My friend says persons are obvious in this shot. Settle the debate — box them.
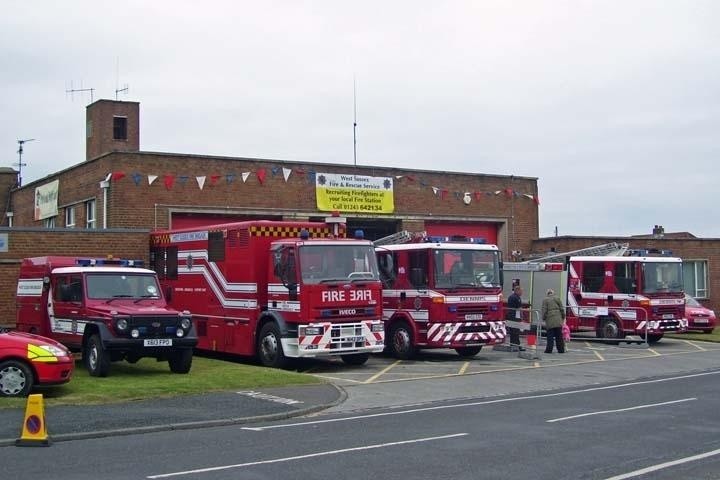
[507,286,532,351]
[542,289,565,353]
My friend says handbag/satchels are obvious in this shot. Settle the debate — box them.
[562,323,570,343]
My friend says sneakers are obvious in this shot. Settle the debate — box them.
[519,348,525,351]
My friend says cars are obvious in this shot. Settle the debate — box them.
[0,326,74,397]
[679,292,716,333]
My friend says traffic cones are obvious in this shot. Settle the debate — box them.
[14,393,53,448]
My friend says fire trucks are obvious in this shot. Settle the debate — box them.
[452,241,684,345]
[374,229,508,358]
[149,215,386,368]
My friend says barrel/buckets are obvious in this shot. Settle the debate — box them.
[526,334,536,344]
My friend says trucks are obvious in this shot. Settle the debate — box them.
[17,252,199,376]
